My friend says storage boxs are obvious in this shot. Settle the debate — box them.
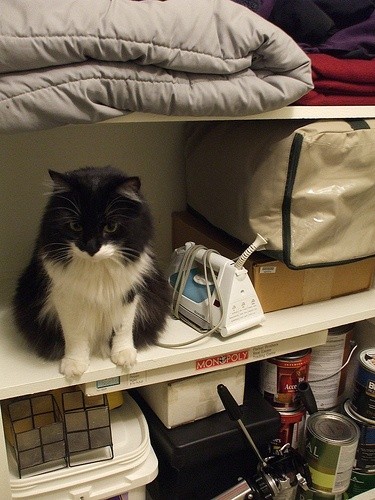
[171,211,375,314]
[136,366,245,429]
[126,389,281,500]
[6,392,159,500]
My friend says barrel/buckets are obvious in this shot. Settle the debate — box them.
[259,329,375,500]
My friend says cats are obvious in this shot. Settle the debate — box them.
[12,164,172,376]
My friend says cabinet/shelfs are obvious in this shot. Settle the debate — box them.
[0,105,375,500]
